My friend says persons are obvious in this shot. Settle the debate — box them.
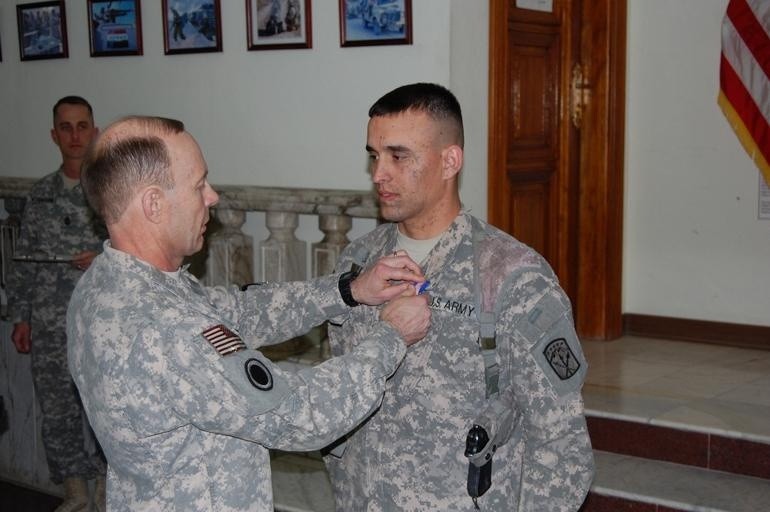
[18,3,298,53]
[62,115,434,511]
[0,94,111,509]
[316,80,596,509]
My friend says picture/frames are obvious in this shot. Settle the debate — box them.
[13,3,69,63]
[338,0,412,48]
[245,0,312,53]
[86,2,146,58]
[161,2,223,55]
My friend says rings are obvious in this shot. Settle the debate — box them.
[77,265,82,268]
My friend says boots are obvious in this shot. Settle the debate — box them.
[54,476,106,512]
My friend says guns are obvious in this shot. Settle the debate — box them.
[464,424,494,499]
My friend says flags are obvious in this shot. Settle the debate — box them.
[716,0,770,188]
